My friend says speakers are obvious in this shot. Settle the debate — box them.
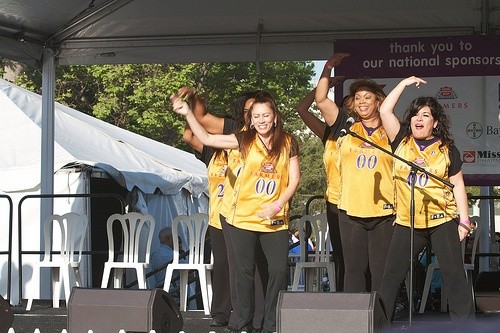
[68,286,184,333]
[0,295,14,333]
[276,289,390,333]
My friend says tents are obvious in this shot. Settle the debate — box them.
[0,0,500,305]
[0,78,209,300]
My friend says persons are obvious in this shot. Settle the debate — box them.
[460,220,470,224]
[170,86,301,333]
[177,236,191,310]
[465,222,477,263]
[146,227,194,305]
[297,53,476,327]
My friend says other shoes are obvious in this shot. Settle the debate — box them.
[209,318,228,326]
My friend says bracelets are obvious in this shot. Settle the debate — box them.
[274,203,281,212]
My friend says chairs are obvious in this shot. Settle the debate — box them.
[162,214,213,316]
[25,211,88,311]
[101,212,155,290]
[405,215,482,314]
[292,212,337,293]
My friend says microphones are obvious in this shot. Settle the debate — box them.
[340,116,355,137]
